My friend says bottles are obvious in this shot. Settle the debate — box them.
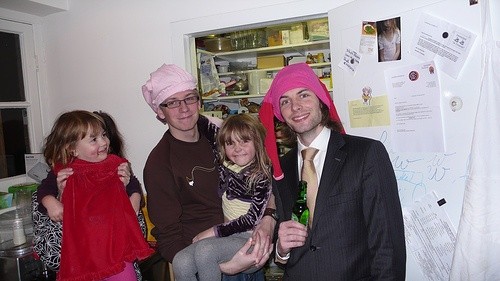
[290,180,310,233]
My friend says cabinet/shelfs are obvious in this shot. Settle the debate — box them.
[197,38,333,100]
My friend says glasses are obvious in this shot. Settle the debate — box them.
[160,96,199,108]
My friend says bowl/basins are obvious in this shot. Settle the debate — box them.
[203,38,232,52]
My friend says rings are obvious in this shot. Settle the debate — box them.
[123,173,127,178]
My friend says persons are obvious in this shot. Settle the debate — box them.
[32,112,148,281]
[270,62,407,281]
[172,114,270,281]
[38,110,155,281]
[143,63,277,281]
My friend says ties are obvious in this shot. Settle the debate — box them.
[301,147,318,230]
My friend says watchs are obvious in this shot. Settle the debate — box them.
[263,206,278,221]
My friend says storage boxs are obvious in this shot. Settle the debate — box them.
[8,183,39,199]
[0,192,13,209]
[266,18,329,44]
[320,78,332,88]
[256,55,286,69]
[259,79,273,94]
[25,153,51,185]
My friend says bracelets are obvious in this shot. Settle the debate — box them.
[274,241,290,261]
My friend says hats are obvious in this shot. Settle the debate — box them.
[142,64,198,113]
[260,63,346,181]
